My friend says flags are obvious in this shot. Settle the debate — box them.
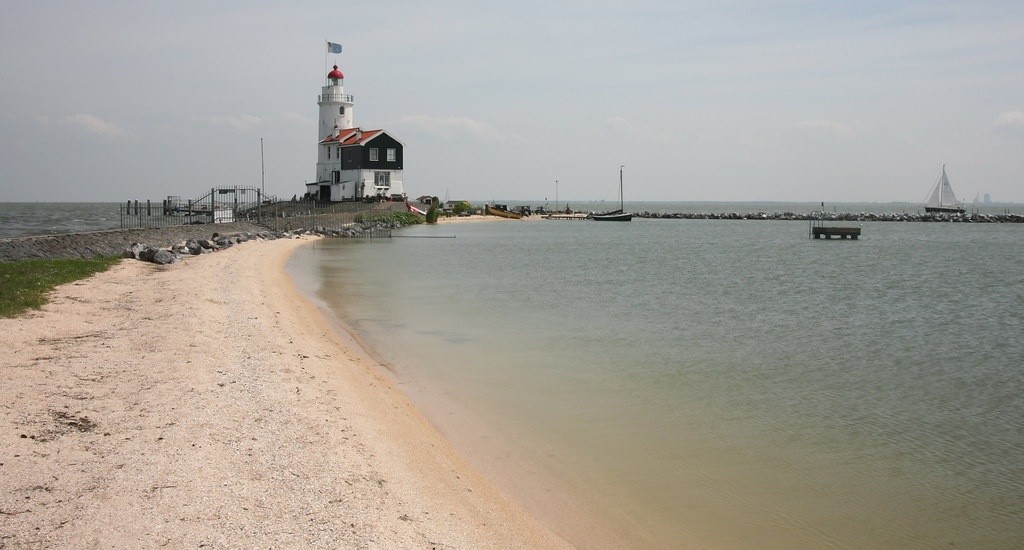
[327,41,343,55]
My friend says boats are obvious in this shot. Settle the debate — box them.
[485,203,522,219]
[593,213,633,223]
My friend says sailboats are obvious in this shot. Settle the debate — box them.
[921,162,965,215]
